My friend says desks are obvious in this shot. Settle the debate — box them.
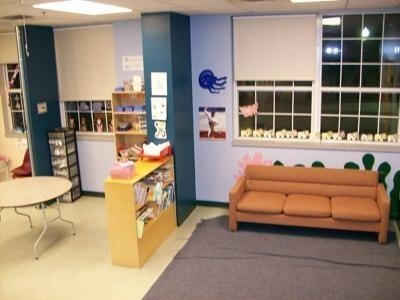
[0,176,76,261]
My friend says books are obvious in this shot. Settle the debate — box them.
[132,164,176,241]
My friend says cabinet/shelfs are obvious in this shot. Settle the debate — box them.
[48,127,84,204]
[63,100,114,133]
[103,91,178,268]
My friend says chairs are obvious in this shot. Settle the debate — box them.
[11,147,32,179]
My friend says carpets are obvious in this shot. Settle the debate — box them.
[140,215,400,300]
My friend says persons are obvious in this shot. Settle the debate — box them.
[204,107,218,138]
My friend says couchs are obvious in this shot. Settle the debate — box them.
[228,163,391,244]
